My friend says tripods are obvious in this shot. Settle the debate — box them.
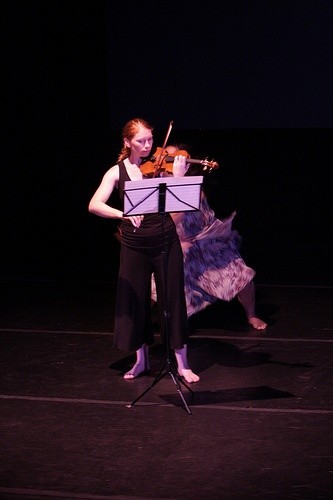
[126,215,200,415]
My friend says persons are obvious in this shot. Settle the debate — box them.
[88,119,201,384]
[150,188,268,331]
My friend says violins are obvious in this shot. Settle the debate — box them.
[139,146,218,179]
